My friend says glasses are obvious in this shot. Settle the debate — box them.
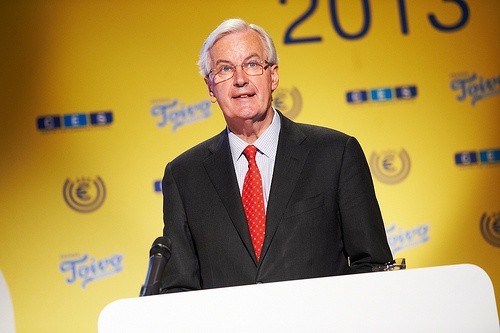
[206,54,274,79]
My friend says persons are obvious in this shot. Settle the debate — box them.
[159,18,394,296]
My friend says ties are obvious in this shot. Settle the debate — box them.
[242,145,266,262]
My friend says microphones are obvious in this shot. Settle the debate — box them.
[140,237,173,297]
